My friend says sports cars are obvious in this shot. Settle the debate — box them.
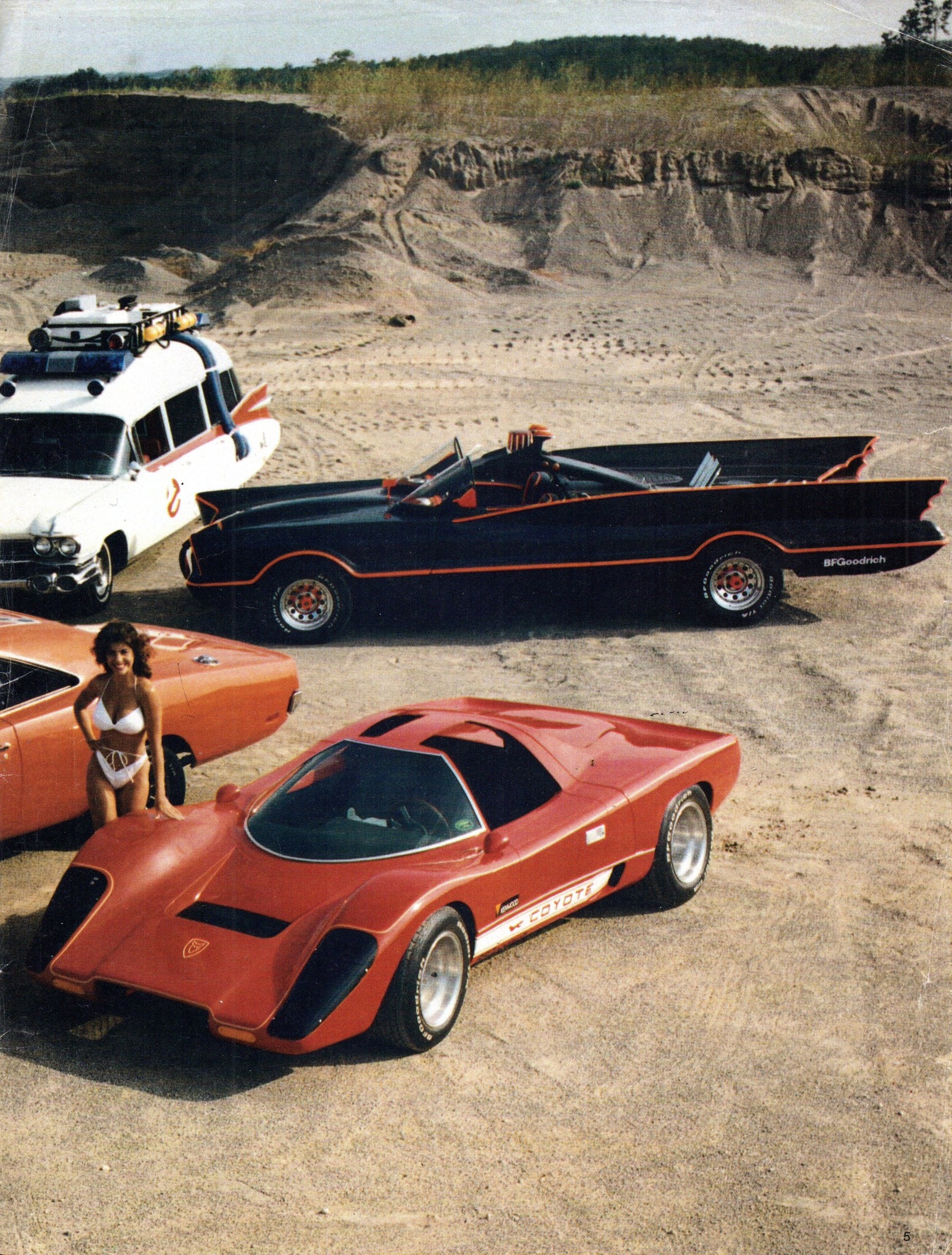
[0,606,299,844]
[26,696,740,1060]
[179,425,949,640]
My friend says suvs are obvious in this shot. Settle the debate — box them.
[1,294,281,608]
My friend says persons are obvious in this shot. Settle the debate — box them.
[73,618,185,830]
